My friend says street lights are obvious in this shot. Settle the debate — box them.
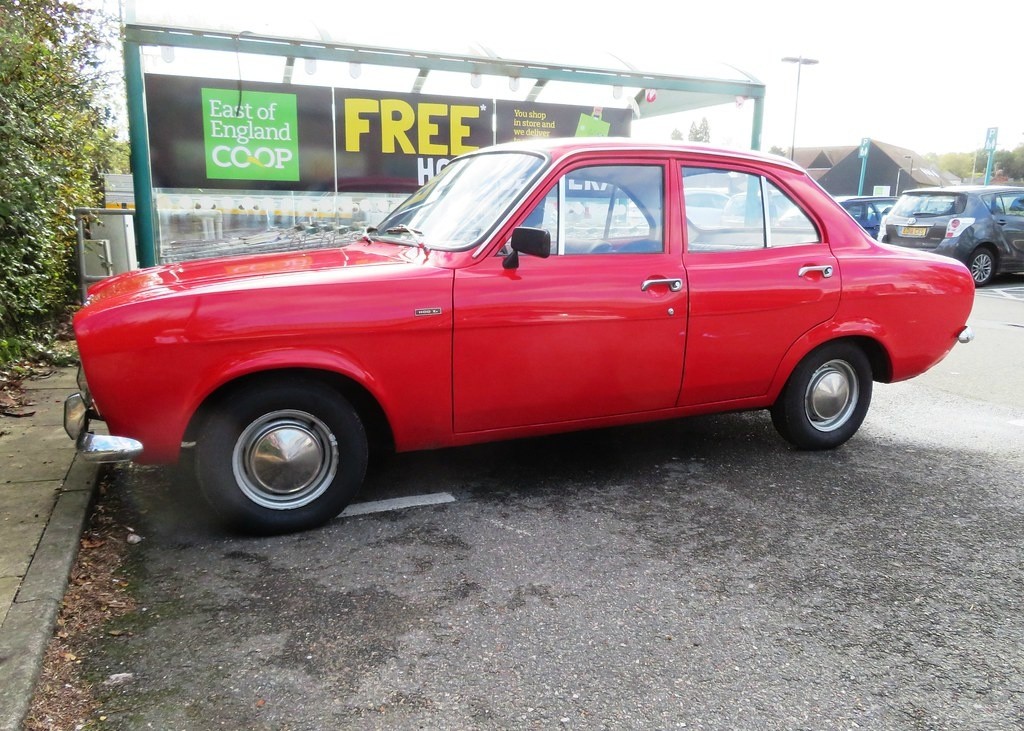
[781,55,820,161]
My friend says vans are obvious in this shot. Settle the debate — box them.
[628,185,1024,286]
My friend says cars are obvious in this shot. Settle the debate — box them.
[62,136,977,537]
